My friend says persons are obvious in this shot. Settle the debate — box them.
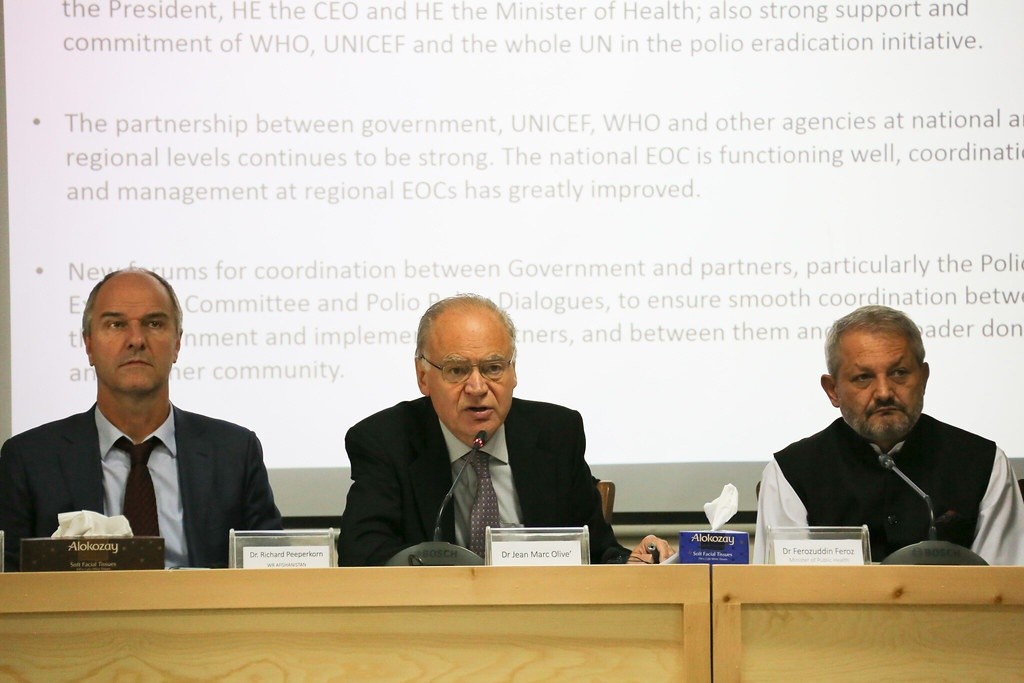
[752,304,1024,566]
[337,293,676,567]
[0,263,286,569]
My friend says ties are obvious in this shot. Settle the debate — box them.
[112,435,164,536]
[462,448,500,559]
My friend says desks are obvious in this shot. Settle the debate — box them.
[0,563,1024,683]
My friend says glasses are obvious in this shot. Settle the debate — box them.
[420,348,516,382]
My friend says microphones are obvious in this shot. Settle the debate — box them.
[878,453,989,567]
[386,431,489,566]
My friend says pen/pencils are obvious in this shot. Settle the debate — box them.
[647,543,660,564]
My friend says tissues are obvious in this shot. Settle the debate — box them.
[19,506,167,573]
[676,479,750,566]
